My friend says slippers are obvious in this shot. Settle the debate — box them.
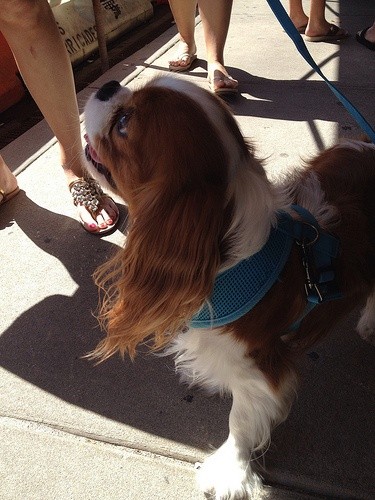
[294,24,306,32]
[66,175,121,236]
[307,24,351,42]
[0,187,22,204]
[171,54,197,71]
[207,70,240,94]
[356,26,375,50]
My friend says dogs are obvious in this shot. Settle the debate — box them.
[82,74,375,500]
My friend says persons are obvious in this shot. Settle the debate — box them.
[356,19,375,51]
[164,1,240,97]
[0,0,119,238]
[287,0,350,42]
